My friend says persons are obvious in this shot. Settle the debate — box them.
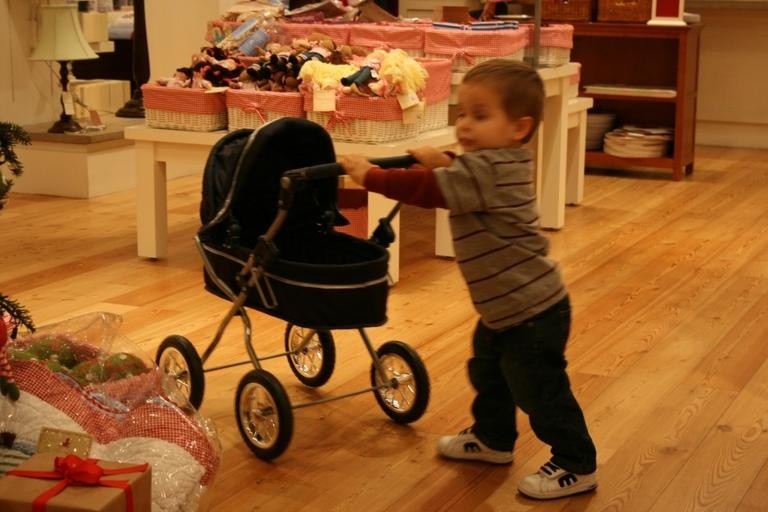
[336,55,599,502]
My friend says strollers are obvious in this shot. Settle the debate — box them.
[154,117,432,462]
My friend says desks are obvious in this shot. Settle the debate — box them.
[1,115,134,199]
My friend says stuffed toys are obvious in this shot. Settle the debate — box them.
[0,315,22,400]
[153,32,431,101]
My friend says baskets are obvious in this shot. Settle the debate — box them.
[141,76,228,132]
[205,18,581,71]
[300,53,454,142]
[224,87,304,133]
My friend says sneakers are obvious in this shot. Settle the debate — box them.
[435,426,514,465]
[518,462,600,501]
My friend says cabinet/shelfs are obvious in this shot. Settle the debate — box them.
[574,19,706,181]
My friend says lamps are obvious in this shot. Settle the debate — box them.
[28,6,99,132]
